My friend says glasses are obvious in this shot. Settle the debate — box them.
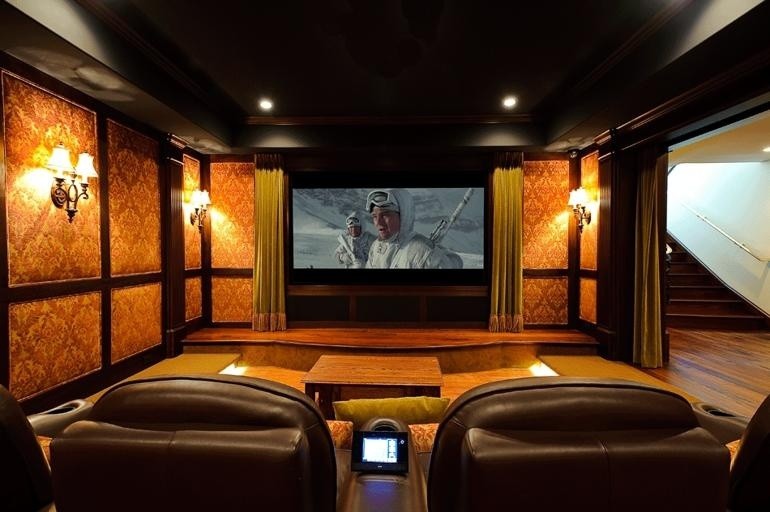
[346,217,360,225]
[365,191,398,211]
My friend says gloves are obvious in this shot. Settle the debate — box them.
[339,252,353,266]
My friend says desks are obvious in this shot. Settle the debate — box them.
[300,353,444,420]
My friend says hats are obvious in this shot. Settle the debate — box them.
[373,192,399,213]
[347,222,360,227]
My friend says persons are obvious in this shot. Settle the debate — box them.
[365,189,457,269]
[335,211,378,269]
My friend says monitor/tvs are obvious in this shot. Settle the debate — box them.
[287,171,492,286]
[350,431,409,475]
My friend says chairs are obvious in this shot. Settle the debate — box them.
[0,374,770,511]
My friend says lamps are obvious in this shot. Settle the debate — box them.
[44,140,99,224]
[565,185,596,234]
[188,186,213,234]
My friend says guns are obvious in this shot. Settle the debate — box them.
[337,234,362,268]
[429,188,475,244]
